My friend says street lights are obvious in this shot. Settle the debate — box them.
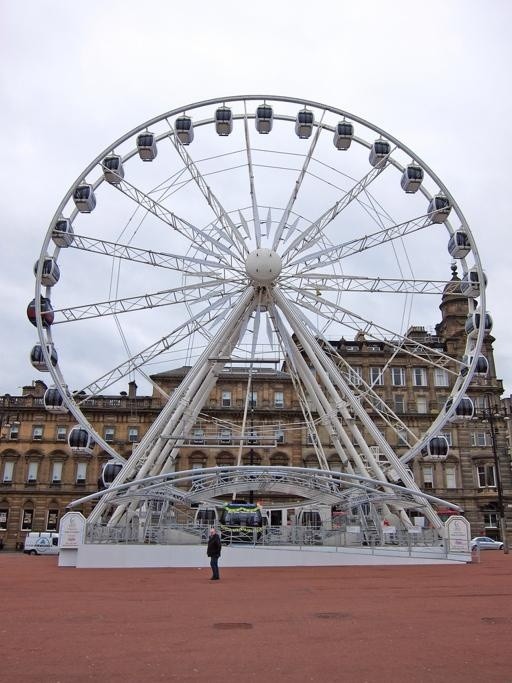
[481,402,511,553]
[250,406,256,465]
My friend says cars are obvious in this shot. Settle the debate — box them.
[472,536,504,550]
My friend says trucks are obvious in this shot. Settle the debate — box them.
[24,531,58,557]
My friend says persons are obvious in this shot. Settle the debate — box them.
[206,527,222,580]
